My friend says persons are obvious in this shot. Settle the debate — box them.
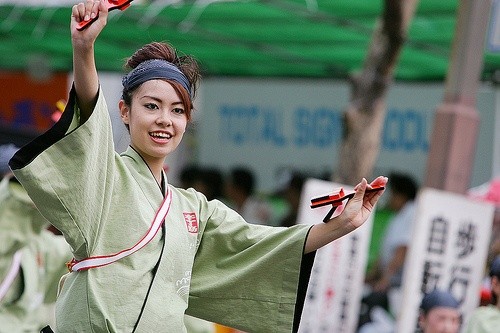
[7,0,389,333]
[419,255,500,333]
[176,160,419,333]
